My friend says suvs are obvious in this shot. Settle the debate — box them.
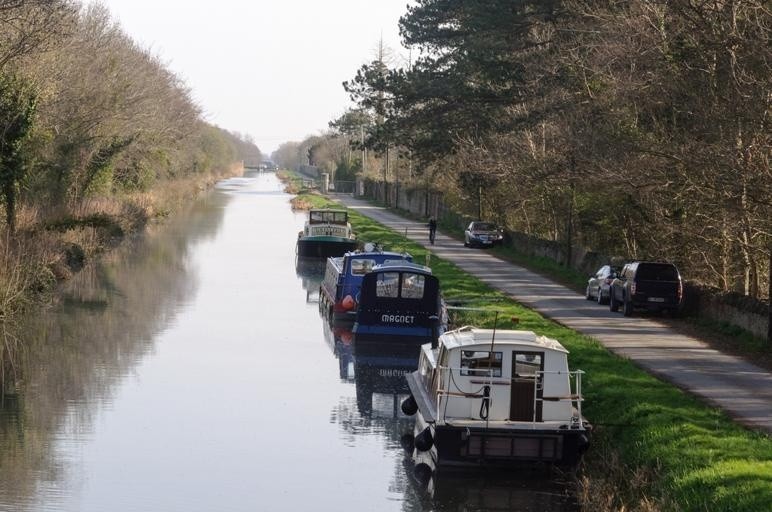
[608,260,686,318]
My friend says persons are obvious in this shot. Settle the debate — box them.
[428,215,437,243]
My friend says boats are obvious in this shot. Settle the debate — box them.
[294,205,358,253]
[403,324,597,473]
[316,234,450,345]
[320,323,425,434]
[294,249,337,305]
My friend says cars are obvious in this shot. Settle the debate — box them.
[462,219,504,249]
[583,262,624,301]
